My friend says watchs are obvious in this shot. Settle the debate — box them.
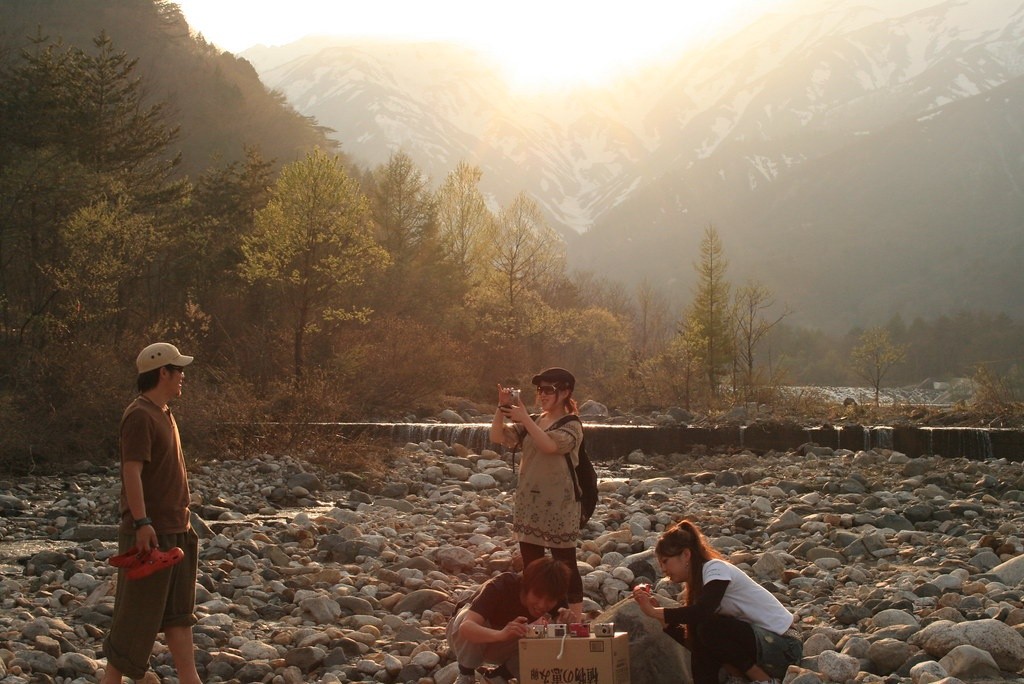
[132,517,152,531]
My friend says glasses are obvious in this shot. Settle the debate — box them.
[537,384,561,395]
[173,367,184,371]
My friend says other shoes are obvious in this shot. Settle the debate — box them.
[748,678,780,684]
[726,676,748,684]
[452,673,475,683]
[480,672,509,684]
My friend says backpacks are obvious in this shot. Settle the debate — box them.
[512,414,599,530]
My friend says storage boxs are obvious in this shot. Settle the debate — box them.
[518,632,631,684]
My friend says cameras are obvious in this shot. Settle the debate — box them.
[594,622,615,637]
[510,389,521,400]
[546,624,567,637]
[639,584,650,593]
[525,625,545,639]
[569,623,591,638]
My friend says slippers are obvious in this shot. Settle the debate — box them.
[110,547,138,568]
[127,547,185,580]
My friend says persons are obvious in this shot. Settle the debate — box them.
[488,368,586,629]
[443,556,577,684]
[632,520,803,684]
[100,342,204,684]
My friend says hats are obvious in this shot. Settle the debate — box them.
[136,342,194,373]
[532,367,575,390]
